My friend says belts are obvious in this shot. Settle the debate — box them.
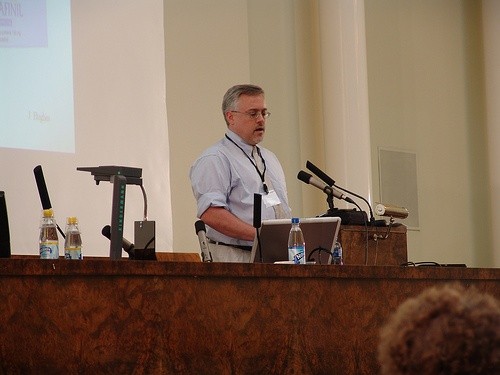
[209,239,252,251]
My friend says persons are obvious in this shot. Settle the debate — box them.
[190,85,291,262]
[378,283,500,375]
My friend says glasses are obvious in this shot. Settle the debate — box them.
[231,111,271,119]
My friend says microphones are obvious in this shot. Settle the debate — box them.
[34,165,66,238]
[297,170,346,200]
[306,160,386,228]
[195,220,212,262]
[253,192,264,263]
[101,225,134,257]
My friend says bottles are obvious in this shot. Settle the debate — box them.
[287,217,306,264]
[333,242,344,265]
[39,210,59,259]
[64,217,83,260]
